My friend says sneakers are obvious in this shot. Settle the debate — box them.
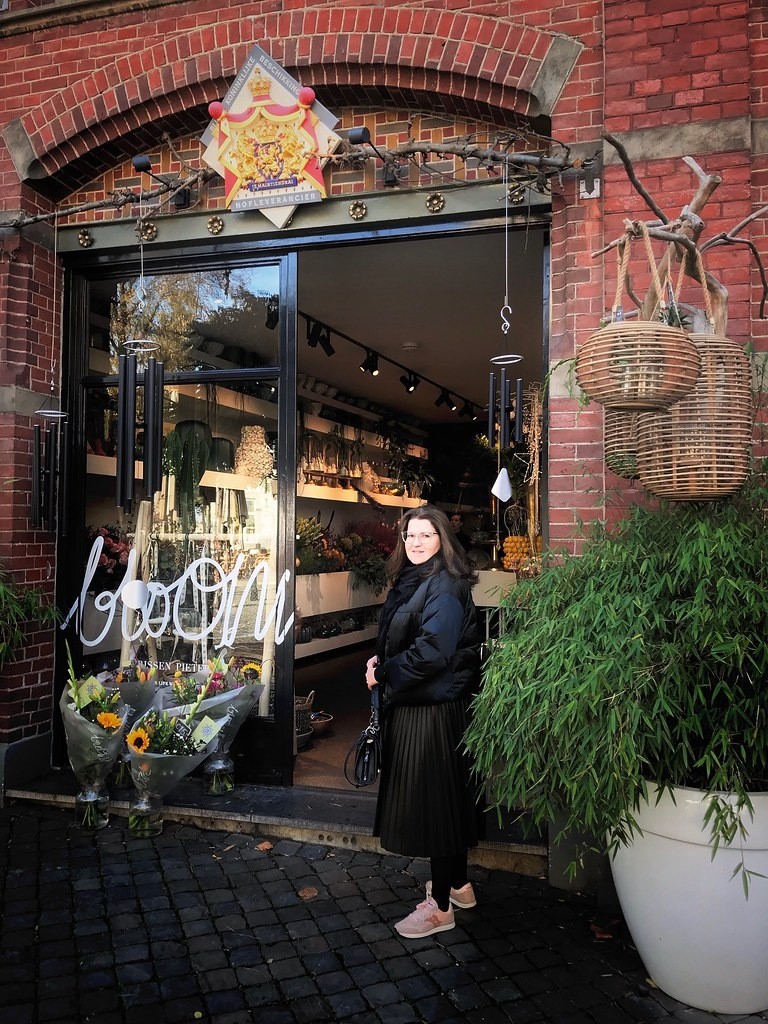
[394,892,455,939]
[425,880,477,909]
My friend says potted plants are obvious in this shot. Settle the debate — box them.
[456,497,768,1015]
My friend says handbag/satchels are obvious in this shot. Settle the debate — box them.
[343,685,386,788]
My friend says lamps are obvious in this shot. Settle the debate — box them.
[266,301,279,331]
[457,400,470,417]
[358,352,379,372]
[398,372,414,392]
[434,395,443,408]
[347,127,402,186]
[467,405,477,421]
[441,392,456,411]
[131,153,189,209]
[306,323,323,351]
[371,364,380,377]
[320,332,334,355]
[405,377,420,394]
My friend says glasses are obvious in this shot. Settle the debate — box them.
[401,531,439,544]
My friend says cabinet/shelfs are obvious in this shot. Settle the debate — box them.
[80,310,534,665]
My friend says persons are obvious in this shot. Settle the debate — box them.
[365,506,478,939]
[450,512,472,554]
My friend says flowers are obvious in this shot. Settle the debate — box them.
[96,660,156,728]
[59,638,124,789]
[160,653,265,753]
[124,704,221,798]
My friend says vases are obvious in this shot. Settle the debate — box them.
[201,753,235,797]
[112,755,132,787]
[127,791,163,838]
[75,778,108,829]
[159,765,193,797]
